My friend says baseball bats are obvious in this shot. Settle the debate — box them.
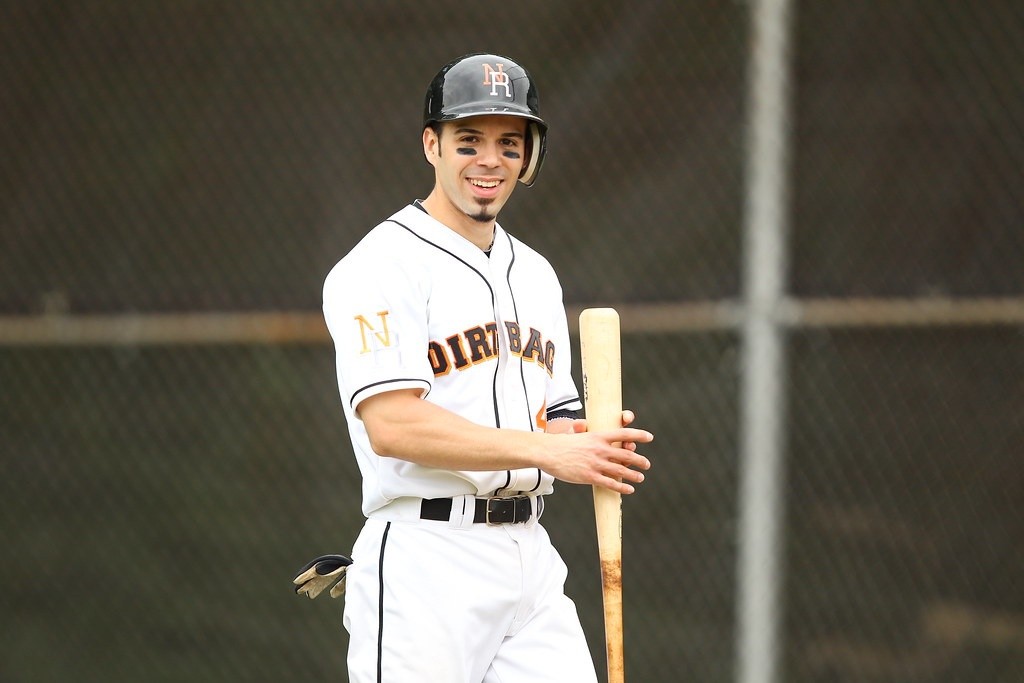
[580,306,626,683]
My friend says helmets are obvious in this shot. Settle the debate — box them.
[421,52,549,188]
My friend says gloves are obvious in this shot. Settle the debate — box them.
[293,555,353,599]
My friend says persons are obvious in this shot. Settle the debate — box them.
[323,54,656,683]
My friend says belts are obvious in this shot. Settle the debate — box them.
[420,496,532,527]
